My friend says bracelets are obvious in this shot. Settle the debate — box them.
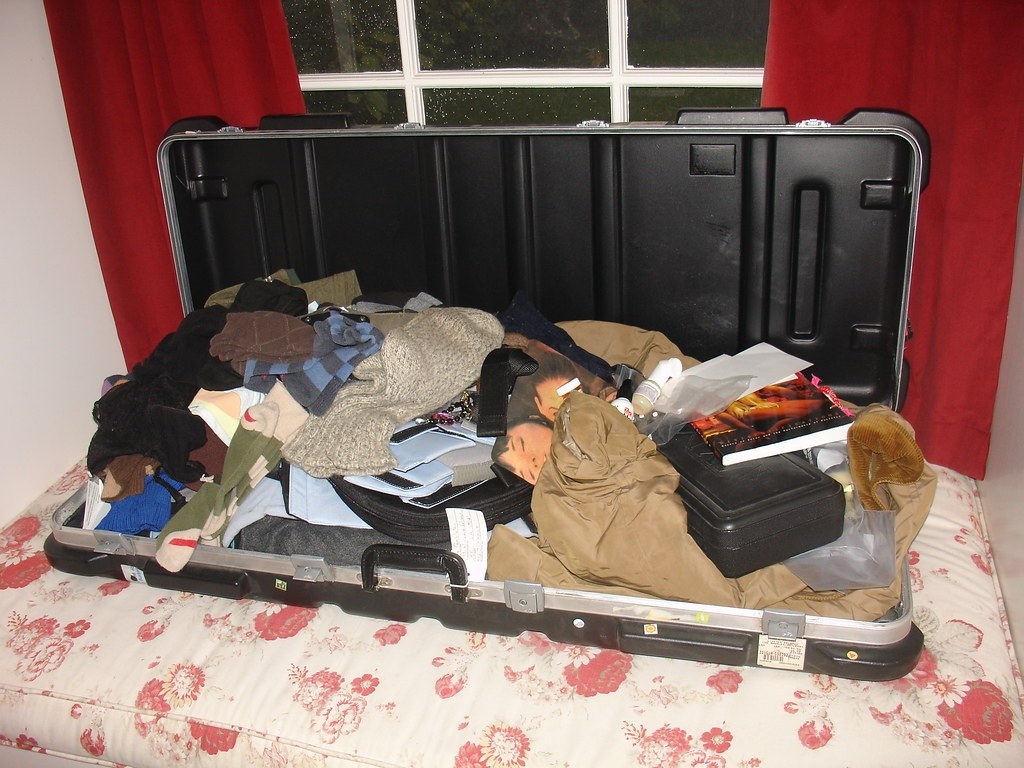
[428,401,469,424]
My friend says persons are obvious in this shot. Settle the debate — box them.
[526,352,584,426]
[715,385,828,443]
[491,414,556,483]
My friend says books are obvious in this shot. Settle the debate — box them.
[688,372,856,465]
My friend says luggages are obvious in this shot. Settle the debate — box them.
[33,103,929,679]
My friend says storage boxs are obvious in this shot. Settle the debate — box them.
[659,426,848,580]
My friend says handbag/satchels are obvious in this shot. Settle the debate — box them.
[328,392,534,546]
